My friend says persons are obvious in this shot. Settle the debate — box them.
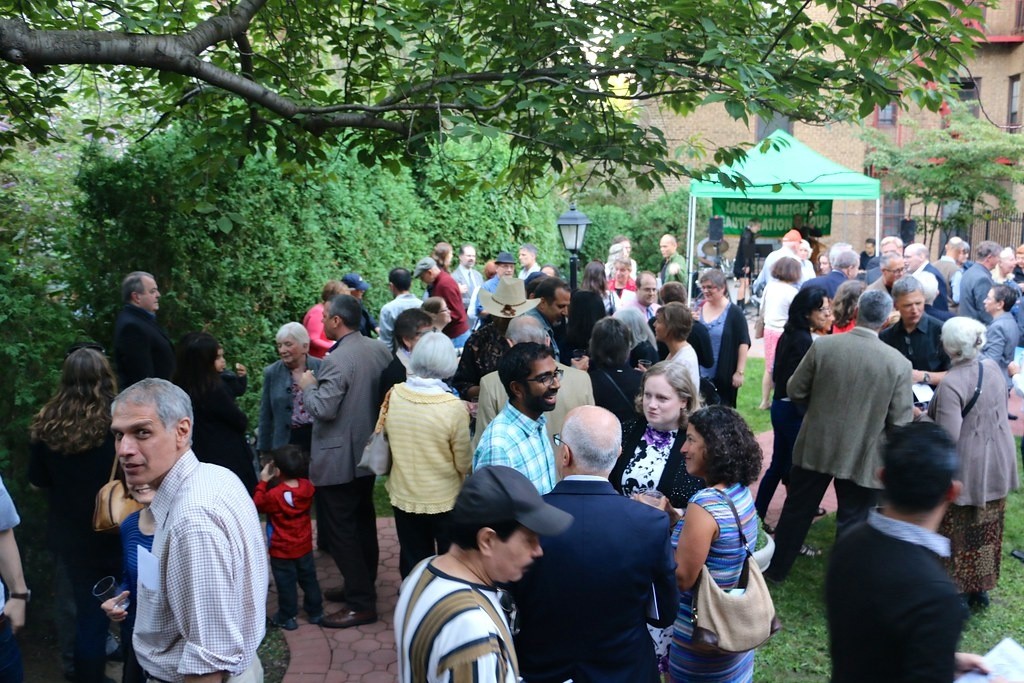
[0,217,1024,683]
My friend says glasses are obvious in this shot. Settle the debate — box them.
[495,586,522,636]
[414,327,437,336]
[817,307,831,312]
[437,307,450,316]
[517,369,565,388]
[553,433,571,452]
[701,284,718,290]
[883,267,904,273]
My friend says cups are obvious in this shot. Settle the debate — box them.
[572,349,586,362]
[92,576,130,613]
[638,359,652,370]
[640,490,663,508]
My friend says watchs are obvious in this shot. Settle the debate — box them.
[922,371,930,384]
[9,589,31,602]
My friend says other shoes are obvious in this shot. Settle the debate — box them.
[816,507,827,516]
[308,611,323,624]
[269,614,300,631]
[759,401,772,410]
[799,545,822,558]
[762,521,776,533]
[1011,550,1024,563]
[762,570,785,585]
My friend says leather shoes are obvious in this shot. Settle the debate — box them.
[324,586,378,603]
[319,606,377,628]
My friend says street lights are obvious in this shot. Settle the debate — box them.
[558,202,593,291]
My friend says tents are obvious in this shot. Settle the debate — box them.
[684,128,880,309]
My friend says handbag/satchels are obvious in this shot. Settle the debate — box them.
[357,388,391,477]
[754,319,764,338]
[93,453,143,534]
[690,488,783,657]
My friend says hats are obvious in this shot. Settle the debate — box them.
[455,465,574,537]
[781,229,804,243]
[341,274,370,290]
[413,256,437,278]
[1016,243,1024,254]
[479,253,541,318]
